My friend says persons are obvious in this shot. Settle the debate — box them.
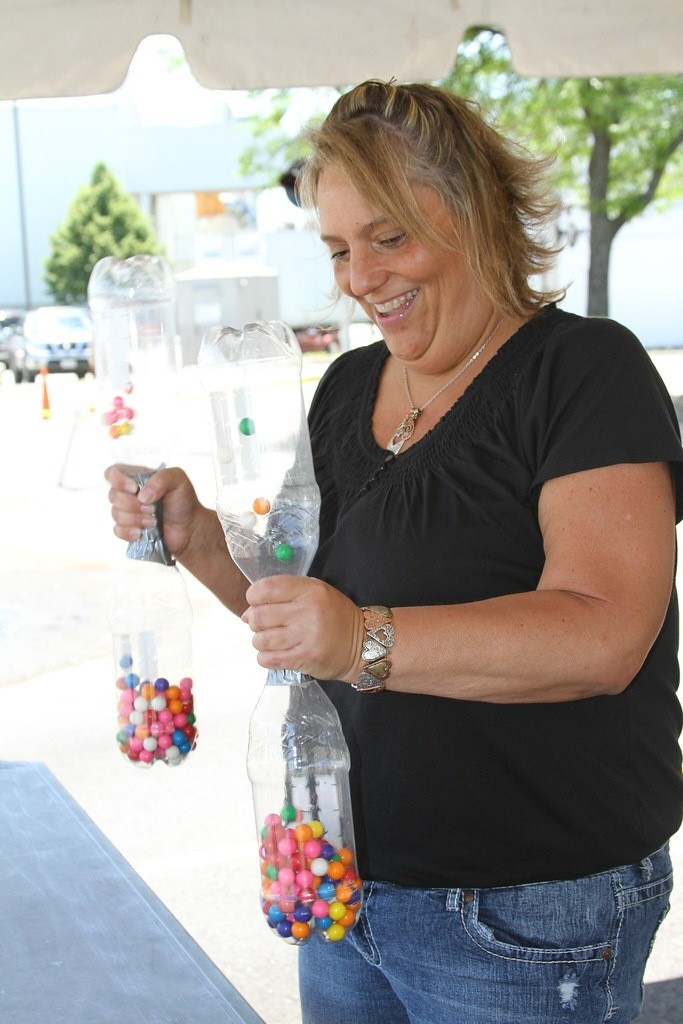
[102,79,683,1024]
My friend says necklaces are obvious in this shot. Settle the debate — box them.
[386,316,507,455]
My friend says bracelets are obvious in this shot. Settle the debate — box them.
[350,605,395,695]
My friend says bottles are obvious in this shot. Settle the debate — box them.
[113,515,198,768]
[197,320,321,586]
[245,667,364,944]
[89,254,189,497]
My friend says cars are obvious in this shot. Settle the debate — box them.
[10,304,96,383]
[0,307,26,371]
